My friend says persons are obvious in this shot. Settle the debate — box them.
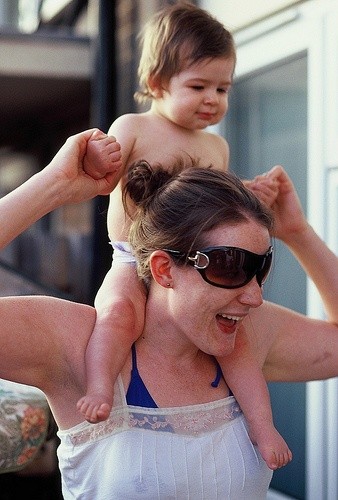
[76,4,293,470]
[0,128,338,500]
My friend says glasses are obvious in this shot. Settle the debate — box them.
[162,245,274,289]
